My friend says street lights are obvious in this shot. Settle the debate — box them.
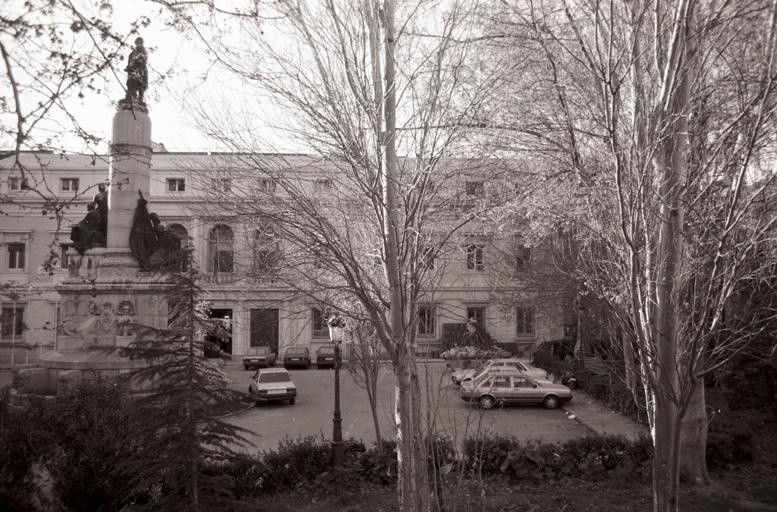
[327,314,346,469]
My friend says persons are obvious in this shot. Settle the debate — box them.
[80,202,102,224]
[124,36,150,105]
[92,182,107,214]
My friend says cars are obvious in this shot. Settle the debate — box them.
[204,341,232,360]
[451,358,573,410]
[315,345,342,368]
[247,368,297,406]
[284,346,311,370]
[242,344,276,371]
[439,345,511,359]
